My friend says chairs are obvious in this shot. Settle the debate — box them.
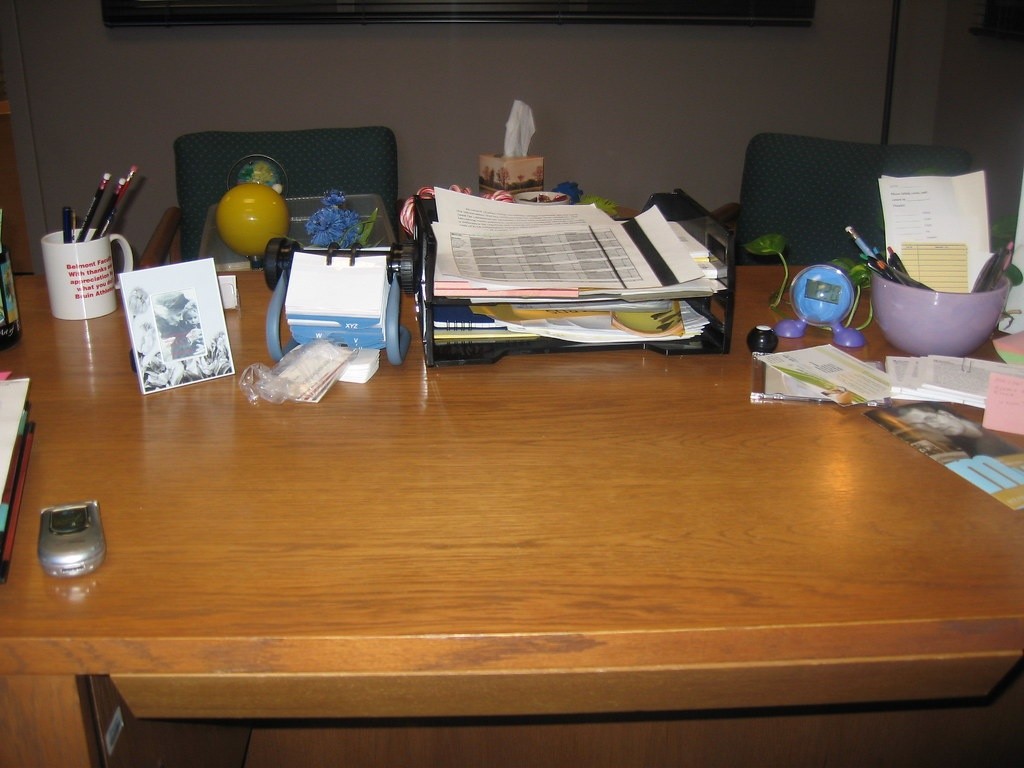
[134,125,407,272]
[737,133,973,266]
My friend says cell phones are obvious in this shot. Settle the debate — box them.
[39,501,105,578]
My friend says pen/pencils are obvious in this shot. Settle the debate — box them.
[62,164,138,244]
[844,226,937,291]
[976,241,1014,292]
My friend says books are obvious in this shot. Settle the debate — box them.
[668,221,717,278]
[432,305,539,338]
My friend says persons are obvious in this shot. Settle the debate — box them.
[899,402,1016,458]
[153,292,200,362]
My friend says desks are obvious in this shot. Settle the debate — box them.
[0,271,1024,768]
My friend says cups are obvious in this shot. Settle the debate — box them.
[41,229,133,321]
[0,246,22,350]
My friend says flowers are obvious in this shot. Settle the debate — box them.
[305,192,382,249]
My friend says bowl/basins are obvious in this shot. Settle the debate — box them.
[514,190,572,205]
[872,271,1011,357]
